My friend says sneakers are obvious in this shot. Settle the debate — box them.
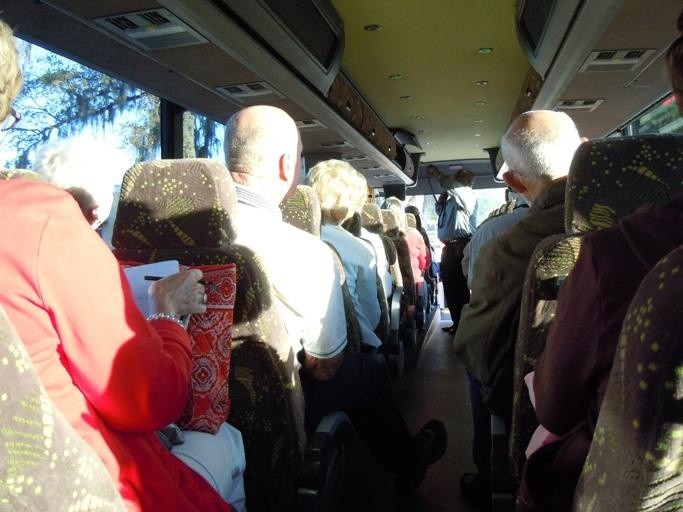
[409,420,449,488]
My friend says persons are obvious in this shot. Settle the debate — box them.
[428,160,482,333]
[447,109,588,509]
[0,16,233,512]
[35,126,141,250]
[512,32,681,510]
[224,106,452,512]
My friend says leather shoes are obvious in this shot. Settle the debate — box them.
[441,325,457,334]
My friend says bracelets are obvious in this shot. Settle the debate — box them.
[148,312,185,328]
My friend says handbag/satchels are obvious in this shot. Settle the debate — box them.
[115,259,238,434]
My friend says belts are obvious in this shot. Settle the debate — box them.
[448,239,461,243]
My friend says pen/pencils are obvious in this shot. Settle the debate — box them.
[144,275,213,285]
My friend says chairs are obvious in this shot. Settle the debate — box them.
[100,157,438,509]
[458,134,682,511]
[0,303,128,511]
